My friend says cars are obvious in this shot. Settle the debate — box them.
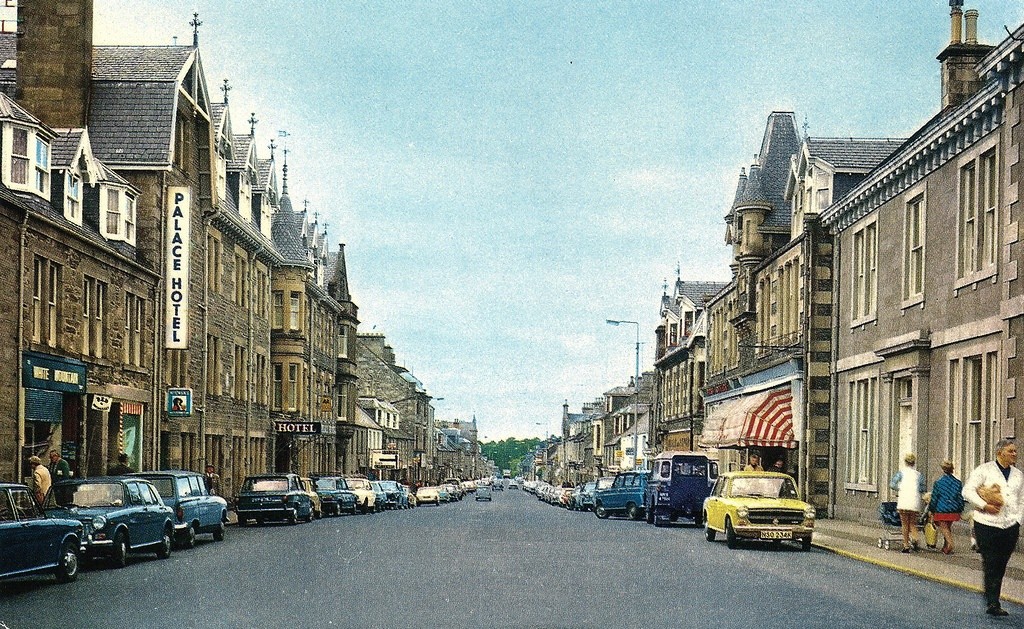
[42,476,176,568]
[300,475,504,519]
[646,451,712,528]
[0,483,86,581]
[508,480,518,489]
[593,470,652,520]
[515,476,616,512]
[703,471,815,552]
[233,472,314,525]
[122,470,231,548]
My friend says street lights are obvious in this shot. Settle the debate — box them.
[537,422,550,450]
[417,396,445,475]
[606,319,640,469]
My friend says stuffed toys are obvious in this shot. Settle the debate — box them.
[973,483,1004,515]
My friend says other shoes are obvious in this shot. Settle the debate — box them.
[901,546,910,553]
[942,546,955,554]
[986,604,1010,616]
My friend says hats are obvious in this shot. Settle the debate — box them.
[205,464,215,469]
[31,456,41,463]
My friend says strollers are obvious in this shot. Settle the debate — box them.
[877,495,924,552]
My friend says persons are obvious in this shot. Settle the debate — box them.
[888,452,925,553]
[961,440,1024,616]
[927,460,964,554]
[27,456,52,504]
[48,449,70,484]
[919,491,932,525]
[202,463,222,496]
[107,453,135,476]
[961,503,981,553]
[744,454,764,471]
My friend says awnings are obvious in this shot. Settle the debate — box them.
[698,387,798,448]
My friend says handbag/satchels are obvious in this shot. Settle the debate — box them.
[924,515,938,547]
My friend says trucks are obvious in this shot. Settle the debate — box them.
[503,470,511,479]
